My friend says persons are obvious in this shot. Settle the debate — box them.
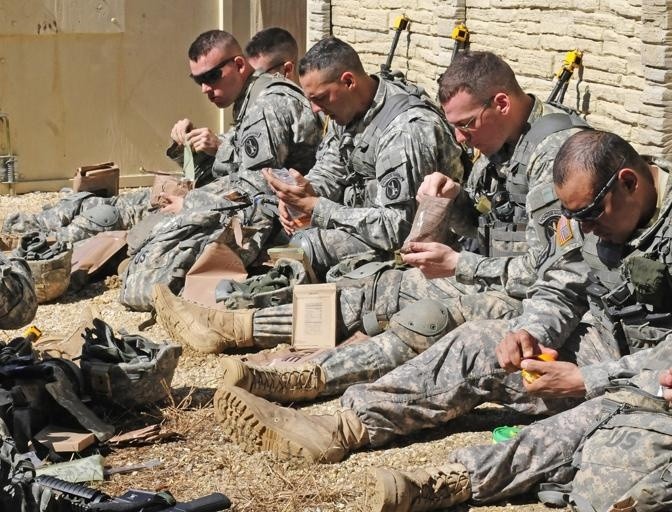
[152,51,598,405]
[212,133,672,511]
[1,28,473,310]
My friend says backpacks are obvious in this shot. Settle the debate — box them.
[563,388,672,512]
[120,211,232,312]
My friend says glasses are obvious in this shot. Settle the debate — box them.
[259,61,286,76]
[444,99,492,130]
[561,159,626,222]
[189,58,237,85]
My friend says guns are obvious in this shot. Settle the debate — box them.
[545,48,582,114]
[380,14,409,80]
[436,22,468,106]
[35,475,231,511]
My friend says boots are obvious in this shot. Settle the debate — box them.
[376,463,471,512]
[150,284,256,354]
[213,358,368,469]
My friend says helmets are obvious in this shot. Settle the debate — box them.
[216,258,307,313]
[0,230,73,330]
[79,317,182,406]
